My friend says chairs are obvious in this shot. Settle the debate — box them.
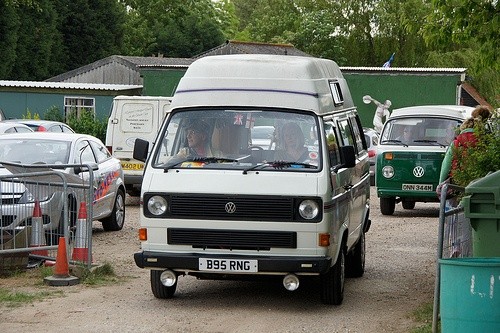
[212,126,250,151]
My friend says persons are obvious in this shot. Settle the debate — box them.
[274,122,313,163]
[446,106,490,153]
[364,96,391,129]
[436,118,488,259]
[394,126,419,145]
[175,120,226,163]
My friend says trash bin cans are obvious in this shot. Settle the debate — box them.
[463,169,500,258]
[438,255,500,332]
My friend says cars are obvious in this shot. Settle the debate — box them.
[0,118,127,247]
[247,121,382,187]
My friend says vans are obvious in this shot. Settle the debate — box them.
[105,95,174,196]
[374,104,477,217]
[132,53,373,306]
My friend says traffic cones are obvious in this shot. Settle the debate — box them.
[42,236,80,286]
[28,200,48,263]
[68,201,99,267]
